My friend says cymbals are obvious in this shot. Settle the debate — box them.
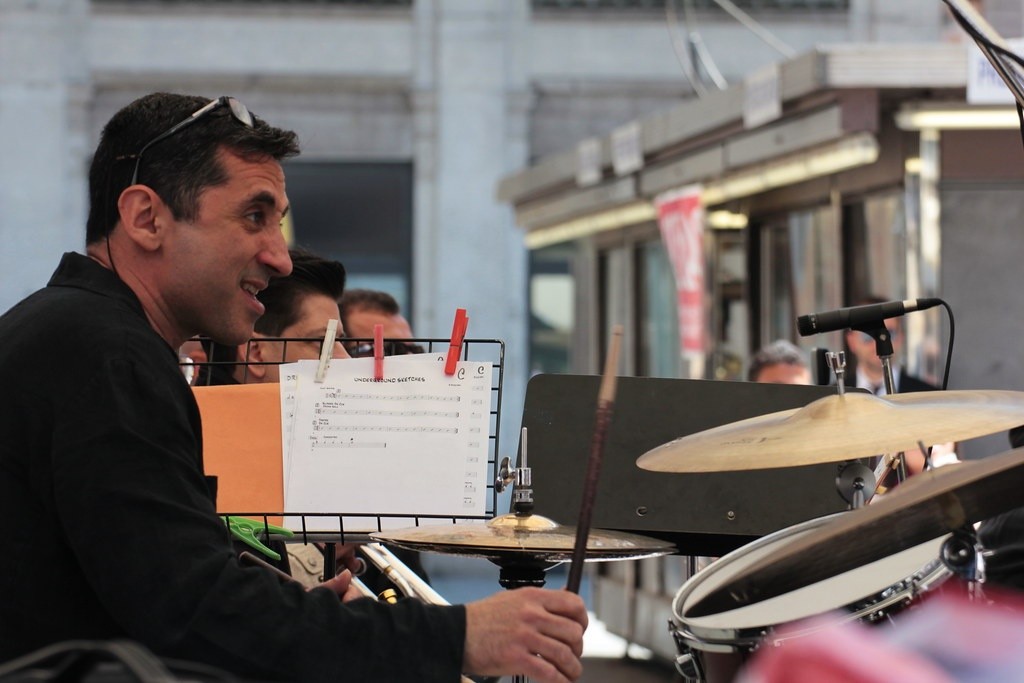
[635,388,1024,473]
[364,514,680,562]
[683,446,1024,619]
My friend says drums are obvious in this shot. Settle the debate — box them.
[666,510,981,683]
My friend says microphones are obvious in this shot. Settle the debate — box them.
[793,295,944,338]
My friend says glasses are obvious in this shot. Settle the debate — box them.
[131,96,255,185]
[345,340,425,357]
[856,330,894,343]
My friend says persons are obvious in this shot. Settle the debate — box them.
[746,295,956,493]
[200,251,355,381]
[339,289,422,357]
[0,95,589,682]
